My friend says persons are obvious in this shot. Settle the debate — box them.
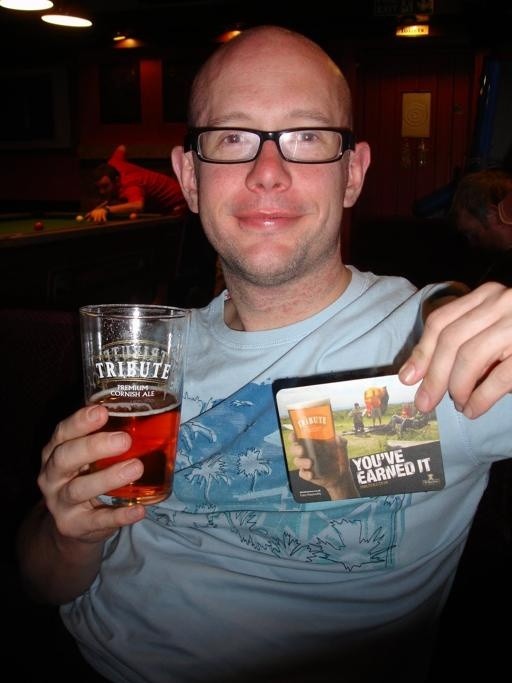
[85,142,219,309]
[288,432,360,500]
[13,24,510,683]
[367,385,429,439]
[349,401,364,435]
[449,170,512,255]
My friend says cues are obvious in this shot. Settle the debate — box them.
[83,199,109,219]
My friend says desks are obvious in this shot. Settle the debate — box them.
[0,212,187,249]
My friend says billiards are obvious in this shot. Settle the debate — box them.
[33,219,44,231]
[74,215,84,224]
[129,212,138,221]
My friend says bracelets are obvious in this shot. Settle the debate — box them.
[101,205,110,218]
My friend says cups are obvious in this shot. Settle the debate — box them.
[80,303,193,507]
[286,398,339,480]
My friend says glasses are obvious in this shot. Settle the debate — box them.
[184,127,354,164]
